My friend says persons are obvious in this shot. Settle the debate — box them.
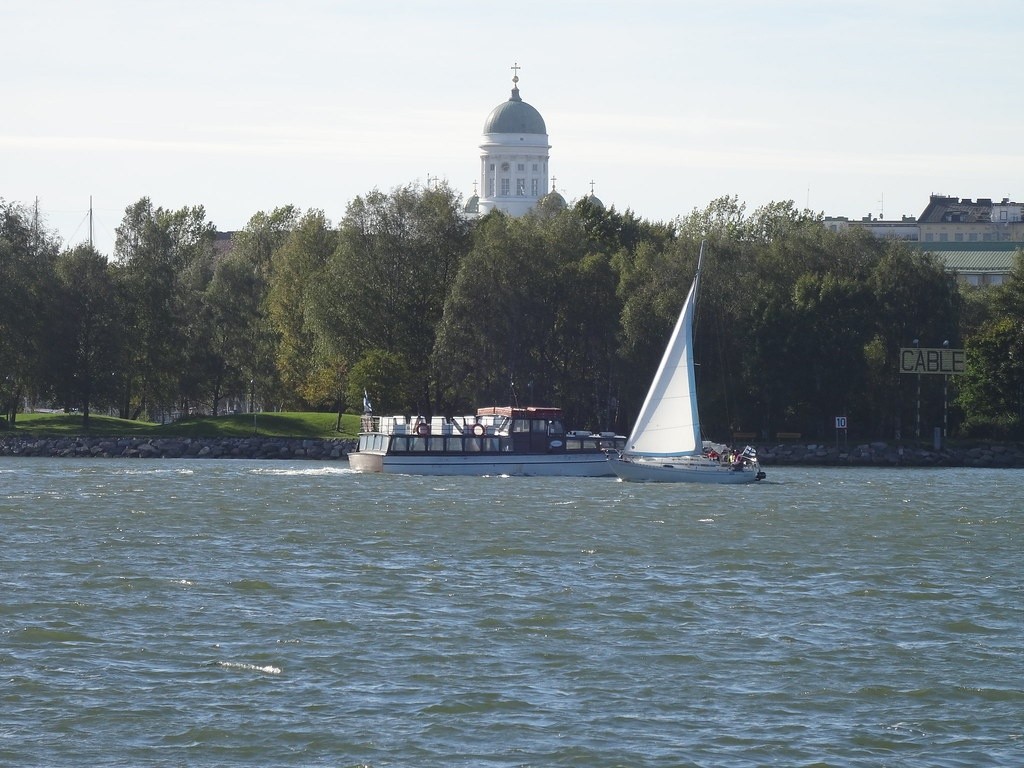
[707,449,745,472]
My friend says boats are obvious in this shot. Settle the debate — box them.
[346,386,627,477]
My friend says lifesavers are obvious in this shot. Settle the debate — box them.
[471,424,486,437]
[416,423,430,436]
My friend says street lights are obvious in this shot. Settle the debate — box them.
[942,340,949,440]
[912,337,920,439]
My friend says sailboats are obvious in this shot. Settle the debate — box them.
[605,238,766,484]
